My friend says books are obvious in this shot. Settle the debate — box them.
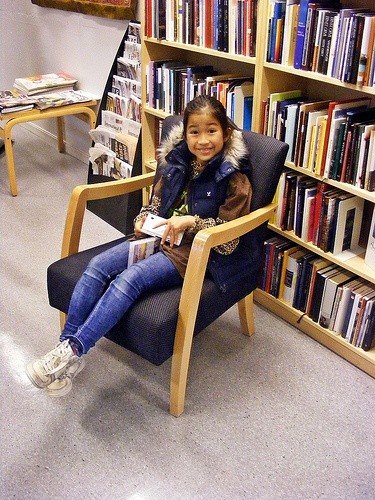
[141,213,185,246]
[87,0,375,353]
[126,236,162,268]
[0,70,103,155]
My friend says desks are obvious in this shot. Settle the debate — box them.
[0,88,100,196]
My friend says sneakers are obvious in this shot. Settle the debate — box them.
[25,339,80,389]
[44,355,86,397]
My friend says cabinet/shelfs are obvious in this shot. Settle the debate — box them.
[141,0,375,378]
[87,20,143,234]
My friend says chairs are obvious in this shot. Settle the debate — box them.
[46,115,290,418]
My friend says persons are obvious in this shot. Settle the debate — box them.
[24,95,253,398]
